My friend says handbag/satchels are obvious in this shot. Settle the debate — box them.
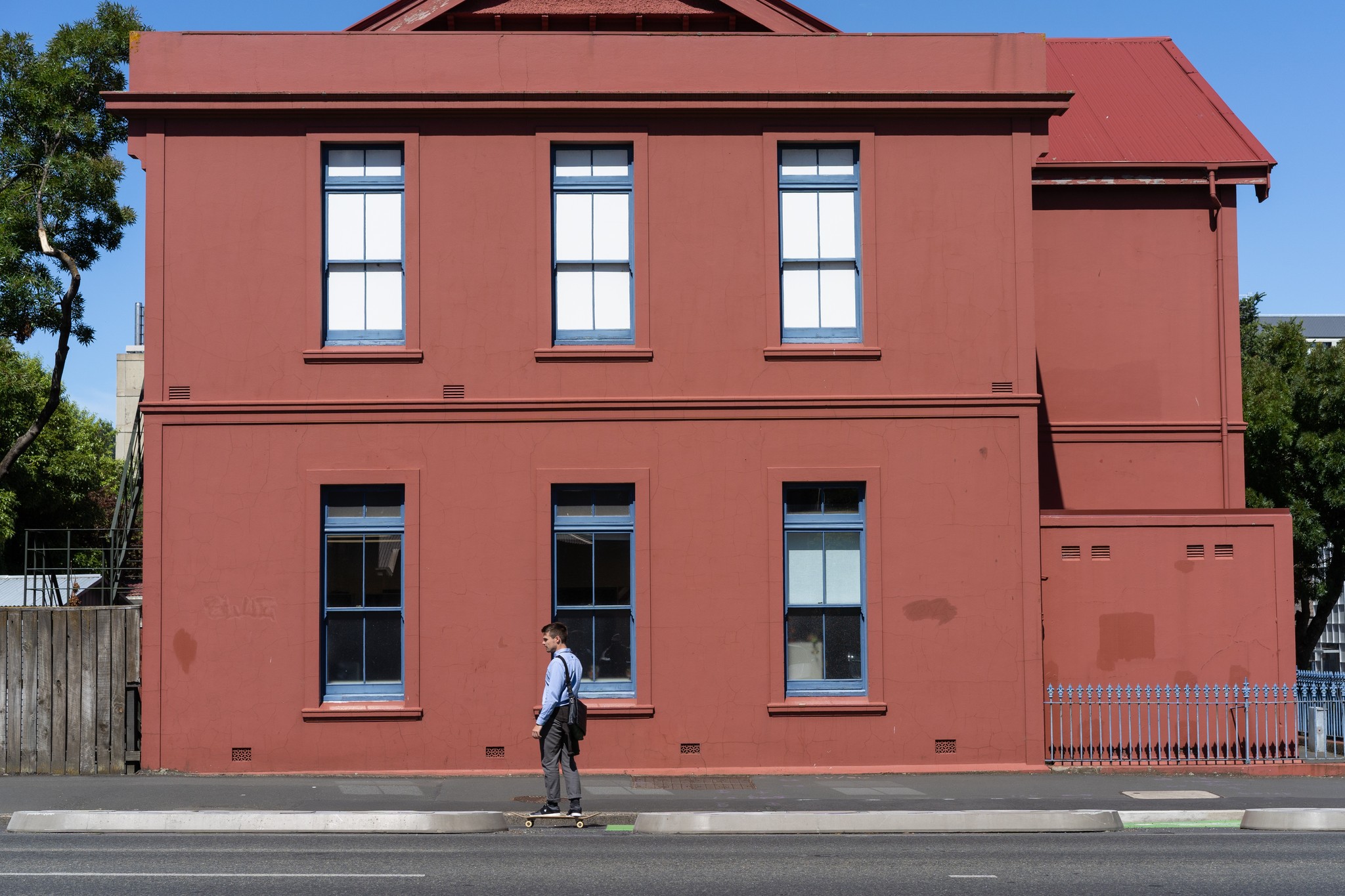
[567,696,587,740]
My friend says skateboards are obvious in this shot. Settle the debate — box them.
[510,810,602,828]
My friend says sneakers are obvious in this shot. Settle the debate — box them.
[529,803,561,816]
[566,805,582,817]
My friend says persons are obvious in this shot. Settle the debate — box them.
[529,623,587,817]
